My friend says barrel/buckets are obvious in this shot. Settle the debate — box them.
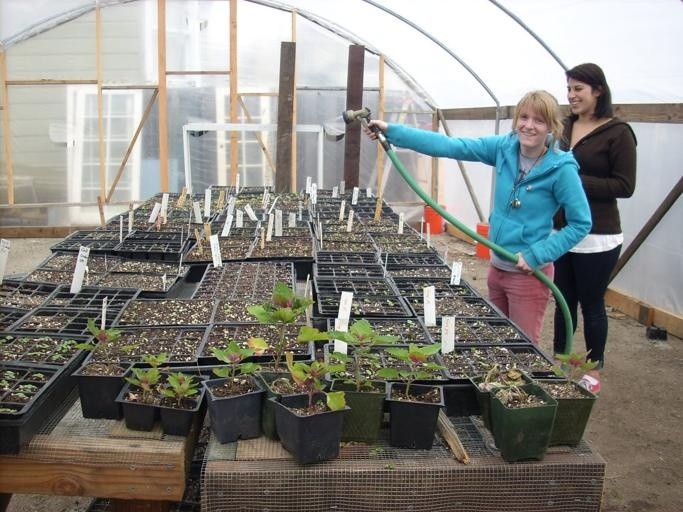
[424,204,446,234]
[475,222,491,260]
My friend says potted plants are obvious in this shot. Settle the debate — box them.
[248,283,444,464]
[1,331,94,455]
[72,317,266,444]
[472,350,597,464]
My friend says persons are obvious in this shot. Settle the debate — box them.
[551,62,637,391]
[364,90,593,349]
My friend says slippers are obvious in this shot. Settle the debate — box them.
[578,374,601,395]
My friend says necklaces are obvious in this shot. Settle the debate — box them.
[511,145,546,208]
